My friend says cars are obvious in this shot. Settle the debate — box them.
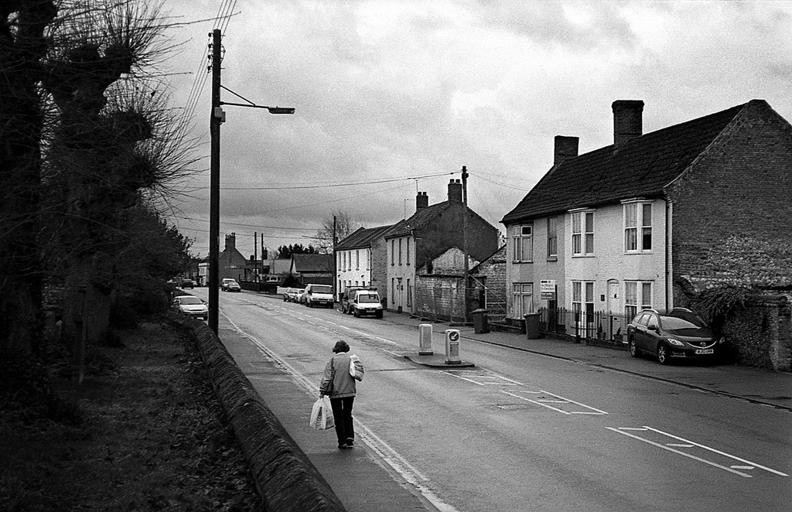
[171,296,209,320]
[283,288,305,302]
[228,283,241,291]
[626,307,726,365]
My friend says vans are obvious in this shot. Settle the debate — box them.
[222,278,235,290]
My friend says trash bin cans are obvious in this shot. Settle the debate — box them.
[469,309,490,334]
[524,312,546,339]
[382,298,387,309]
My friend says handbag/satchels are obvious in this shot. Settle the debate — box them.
[324,382,334,395]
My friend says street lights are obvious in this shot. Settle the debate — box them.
[208,29,295,337]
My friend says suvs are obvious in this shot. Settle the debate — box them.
[181,278,193,289]
[341,286,383,318]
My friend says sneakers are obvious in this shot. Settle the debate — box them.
[338,437,354,449]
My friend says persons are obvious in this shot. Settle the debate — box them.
[318,338,358,450]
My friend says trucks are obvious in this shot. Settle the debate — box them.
[301,284,334,308]
